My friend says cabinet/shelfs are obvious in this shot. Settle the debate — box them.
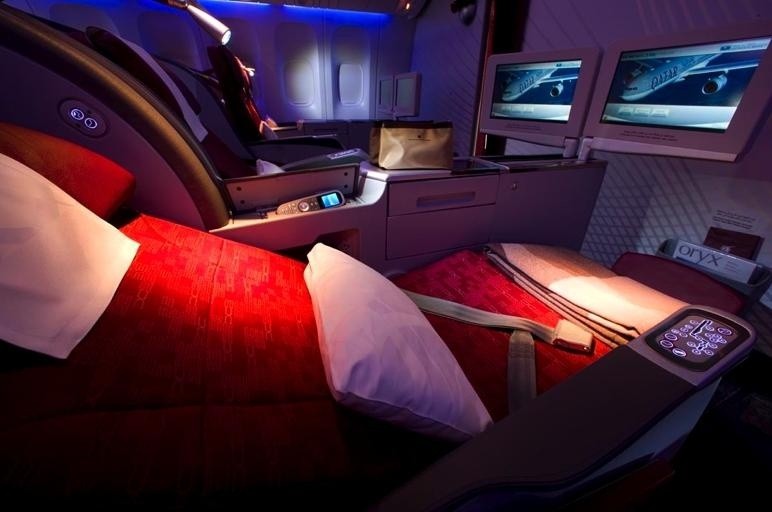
[387,167,604,282]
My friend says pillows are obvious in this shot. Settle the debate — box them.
[305,242,495,445]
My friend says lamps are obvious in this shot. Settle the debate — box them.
[167,0,232,46]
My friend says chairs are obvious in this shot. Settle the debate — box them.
[1,119,753,511]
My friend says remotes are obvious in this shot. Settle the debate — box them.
[276,189,346,218]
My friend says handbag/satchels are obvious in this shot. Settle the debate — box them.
[379,119,455,170]
[370,120,434,165]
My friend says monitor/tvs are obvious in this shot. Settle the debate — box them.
[376,75,394,115]
[479,46,603,148]
[394,71,422,117]
[583,19,772,161]
